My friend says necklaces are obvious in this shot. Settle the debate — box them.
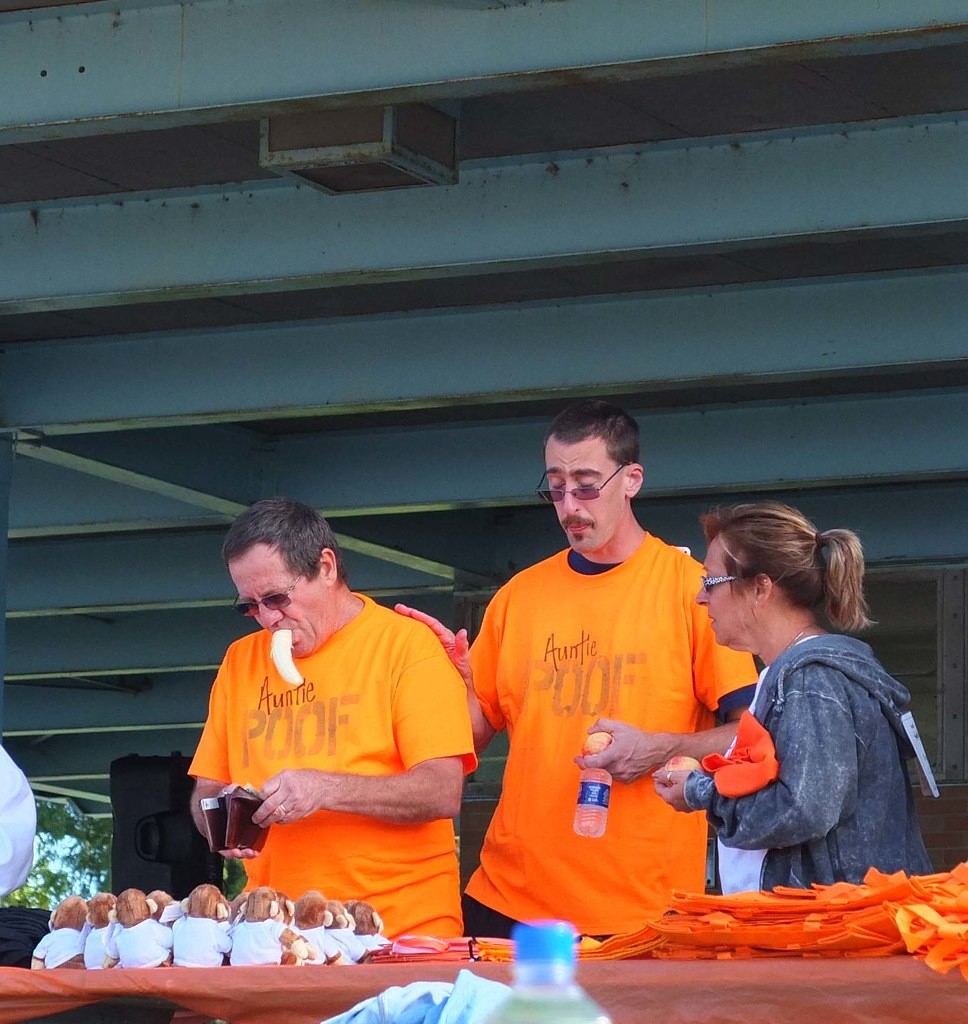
[788,623,815,647]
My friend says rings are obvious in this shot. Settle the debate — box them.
[667,771,673,786]
[280,804,288,813]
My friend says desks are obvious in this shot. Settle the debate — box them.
[0,952,968,1024]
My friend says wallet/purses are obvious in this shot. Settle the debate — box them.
[198,785,265,852]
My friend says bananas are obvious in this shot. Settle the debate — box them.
[270,629,303,685]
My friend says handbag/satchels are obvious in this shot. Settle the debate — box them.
[371,862,968,975]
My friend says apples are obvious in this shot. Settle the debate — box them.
[582,731,613,756]
[664,755,703,786]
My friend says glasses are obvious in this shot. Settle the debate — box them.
[232,571,305,617]
[535,461,634,503]
[701,574,779,592]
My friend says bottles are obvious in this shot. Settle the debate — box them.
[485,919,612,1024]
[573,755,612,838]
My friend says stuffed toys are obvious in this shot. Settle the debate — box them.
[32,884,391,969]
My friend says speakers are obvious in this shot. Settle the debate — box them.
[109,752,224,900]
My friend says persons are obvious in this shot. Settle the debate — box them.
[652,504,936,896]
[188,500,479,937]
[0,745,36,906]
[395,400,760,940]
